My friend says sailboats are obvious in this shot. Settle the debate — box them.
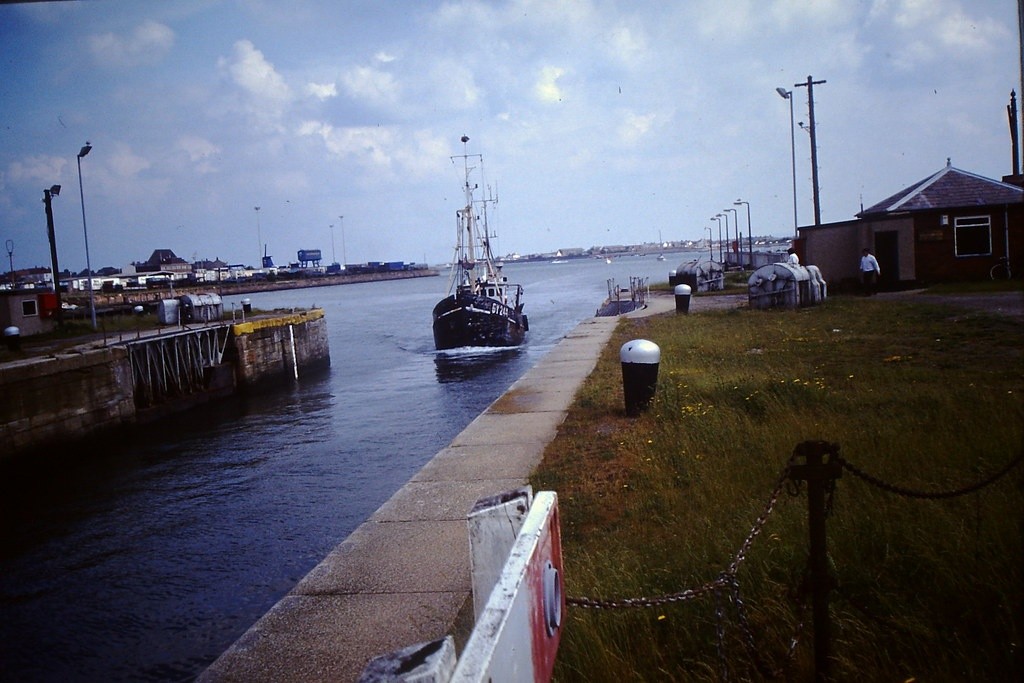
[657,230,665,261]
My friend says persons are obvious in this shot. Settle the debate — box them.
[860,249,881,296]
[788,248,799,264]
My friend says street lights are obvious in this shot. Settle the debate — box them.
[254,205,263,263]
[329,223,336,263]
[338,215,347,264]
[75,141,98,330]
[704,198,753,270]
[777,87,798,238]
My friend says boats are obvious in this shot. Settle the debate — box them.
[551,259,569,264]
[431,129,530,351]
[605,258,611,264]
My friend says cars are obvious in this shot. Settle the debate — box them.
[0,259,421,296]
